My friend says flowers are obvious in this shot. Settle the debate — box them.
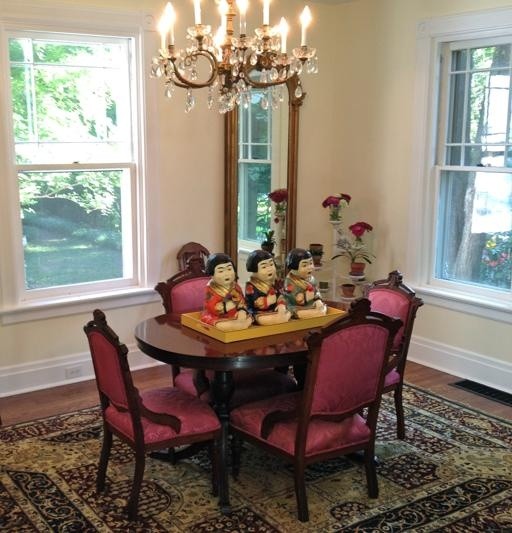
[349,221,373,249]
[319,193,351,221]
[268,188,287,217]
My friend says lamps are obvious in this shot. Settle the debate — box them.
[148,0,319,113]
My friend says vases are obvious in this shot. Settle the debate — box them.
[328,204,343,225]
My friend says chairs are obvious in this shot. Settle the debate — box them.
[83,306,232,521]
[292,268,424,441]
[152,255,297,476]
[230,296,405,521]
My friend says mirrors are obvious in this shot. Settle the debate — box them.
[223,73,300,279]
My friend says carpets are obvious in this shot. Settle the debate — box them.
[0,371,512,533]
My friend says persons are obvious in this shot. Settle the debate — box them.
[201,253,256,332]
[282,248,327,319]
[244,249,292,325]
